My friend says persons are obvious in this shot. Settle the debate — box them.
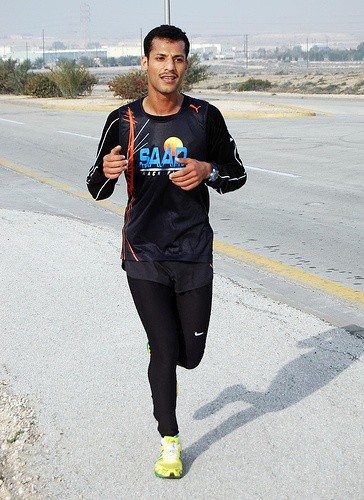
[84,23,249,482]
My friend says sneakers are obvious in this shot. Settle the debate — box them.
[155,433,183,479]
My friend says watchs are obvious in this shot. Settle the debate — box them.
[206,162,219,184]
